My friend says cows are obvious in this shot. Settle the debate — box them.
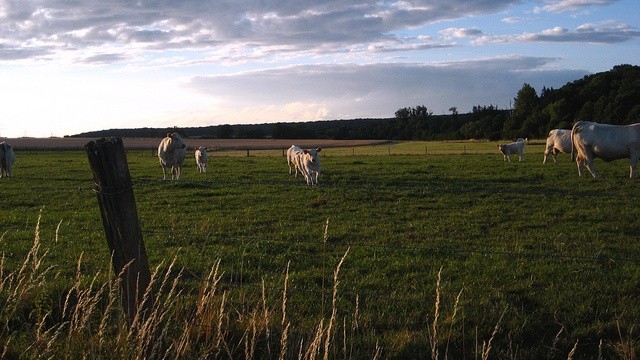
[299,148,322,185]
[543,129,577,164]
[0,141,16,179]
[158,132,186,180]
[287,145,307,178]
[497,142,524,162]
[571,121,640,179]
[195,146,208,173]
